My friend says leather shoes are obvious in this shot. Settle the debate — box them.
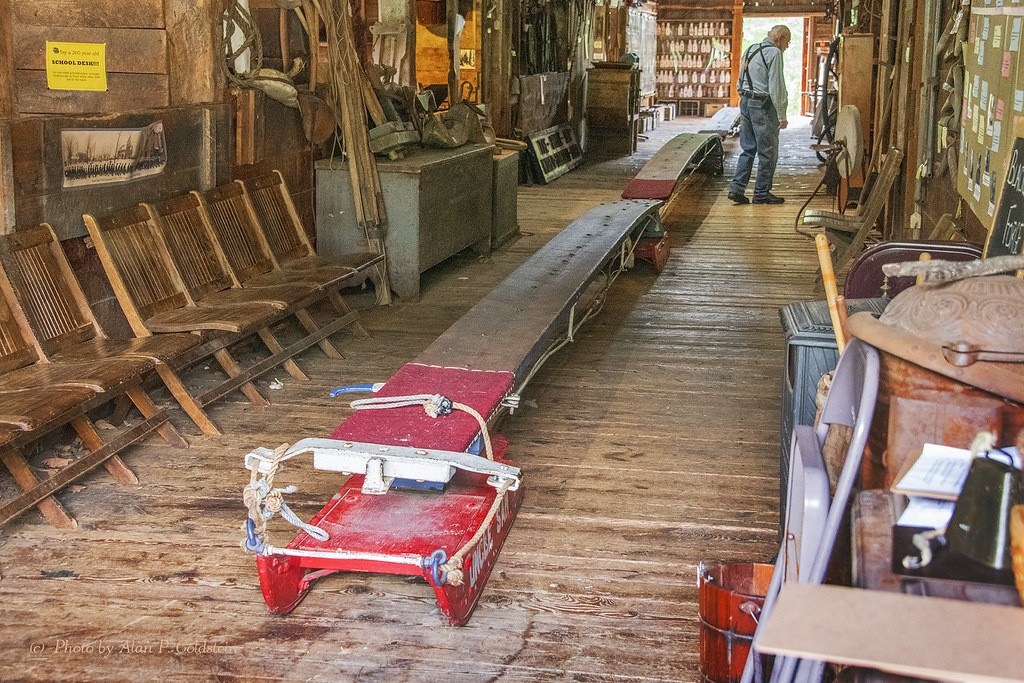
[752,192,785,203]
[727,192,749,203]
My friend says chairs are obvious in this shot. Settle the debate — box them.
[1,168,366,531]
[741,335,882,681]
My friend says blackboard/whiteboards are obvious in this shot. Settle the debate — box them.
[978,136,1024,280]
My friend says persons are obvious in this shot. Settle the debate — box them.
[728,25,791,205]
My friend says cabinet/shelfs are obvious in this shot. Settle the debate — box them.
[585,66,644,155]
[492,149,522,253]
[312,143,496,301]
[840,28,875,188]
[656,18,731,101]
